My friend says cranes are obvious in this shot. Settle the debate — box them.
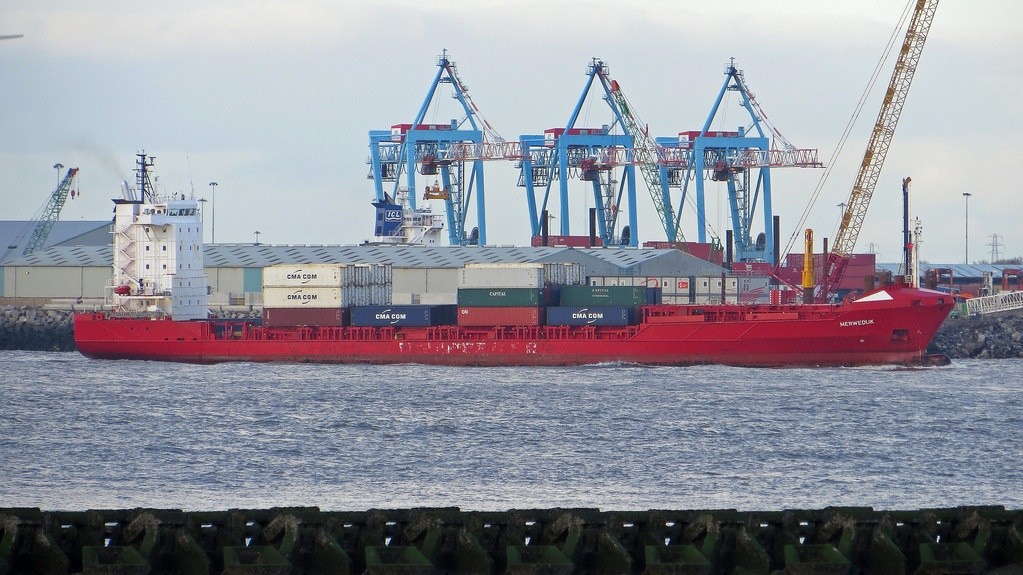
[513,54,829,267]
[363,47,532,247]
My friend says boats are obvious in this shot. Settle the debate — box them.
[113,284,131,295]
[66,151,960,367]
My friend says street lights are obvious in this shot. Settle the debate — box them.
[208,180,219,243]
[252,230,262,243]
[962,190,973,264]
[53,164,64,220]
[835,203,848,252]
[197,197,208,226]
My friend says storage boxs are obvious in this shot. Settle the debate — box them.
[245,263,662,328]
[788,254,876,290]
[678,132,739,149]
[641,241,771,276]
[531,235,602,246]
[769,290,796,305]
[544,128,603,147]
[390,123,451,143]
[206,290,233,306]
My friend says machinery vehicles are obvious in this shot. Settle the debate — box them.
[5,162,82,255]
[762,0,941,304]
